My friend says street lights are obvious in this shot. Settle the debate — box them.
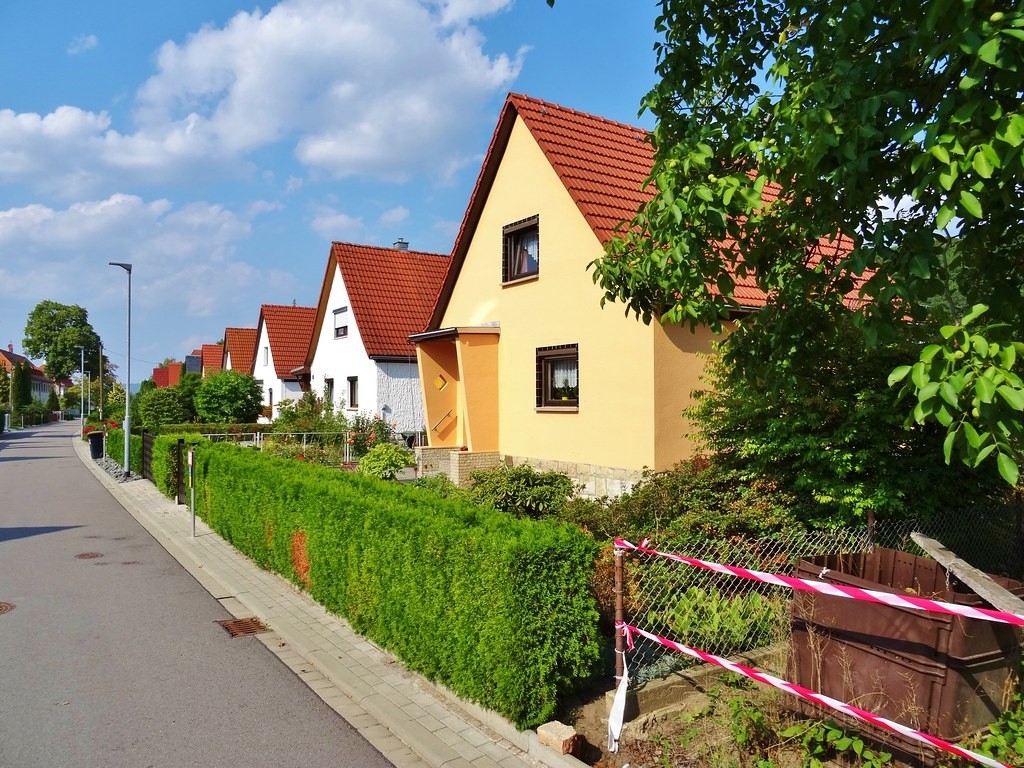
[84,371,90,415]
[73,345,84,426]
[110,262,130,475]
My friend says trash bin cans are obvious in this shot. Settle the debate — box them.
[86,431,103,459]
[781,545,1024,767]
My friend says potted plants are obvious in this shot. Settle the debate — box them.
[559,379,573,400]
